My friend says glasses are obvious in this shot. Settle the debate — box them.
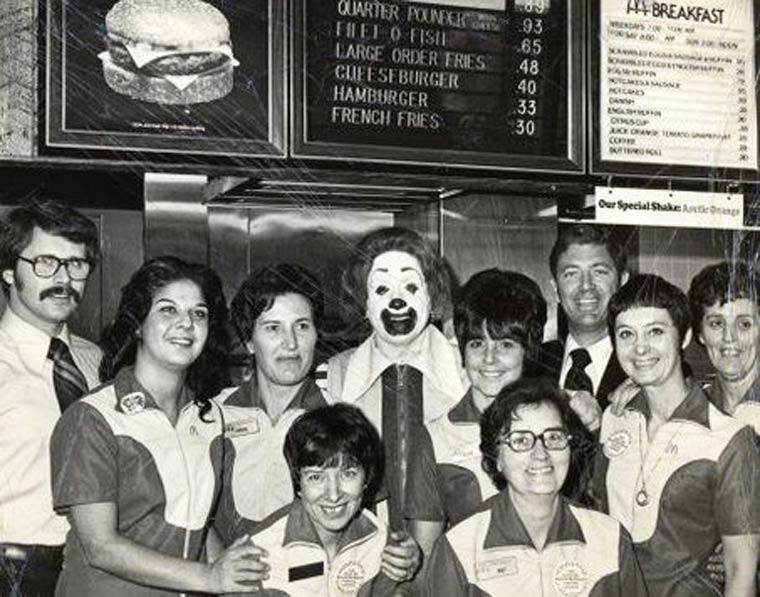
[496,428,574,452]
[18,254,96,281]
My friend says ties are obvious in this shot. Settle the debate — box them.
[564,349,593,396]
[46,338,89,416]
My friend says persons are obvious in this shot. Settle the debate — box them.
[47,255,272,597]
[0,204,105,597]
[206,224,760,597]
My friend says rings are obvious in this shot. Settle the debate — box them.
[406,570,414,581]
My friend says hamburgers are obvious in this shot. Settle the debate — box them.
[97,0,240,104]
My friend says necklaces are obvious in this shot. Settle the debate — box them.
[635,414,683,507]
